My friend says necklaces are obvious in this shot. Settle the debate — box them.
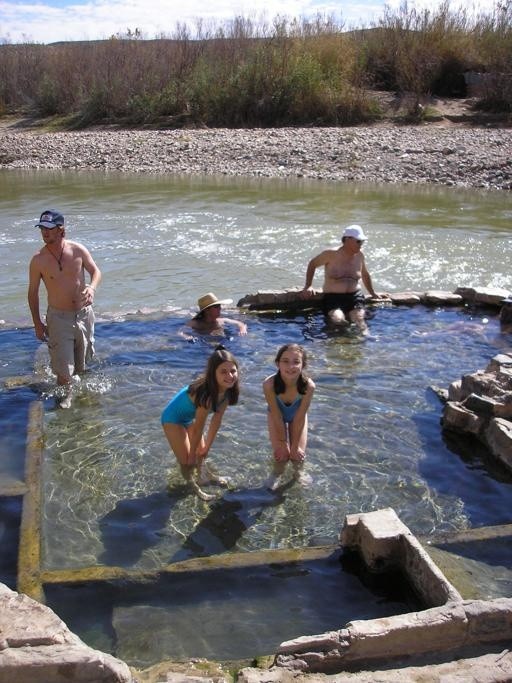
[45,242,64,271]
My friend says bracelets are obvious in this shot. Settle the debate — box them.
[85,284,96,292]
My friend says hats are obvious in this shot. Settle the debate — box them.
[34,209,65,230]
[342,224,369,242]
[191,292,233,320]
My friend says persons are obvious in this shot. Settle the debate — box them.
[161,349,239,501]
[27,209,101,410]
[262,343,315,464]
[299,225,389,336]
[178,292,247,344]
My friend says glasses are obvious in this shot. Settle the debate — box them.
[344,237,362,243]
